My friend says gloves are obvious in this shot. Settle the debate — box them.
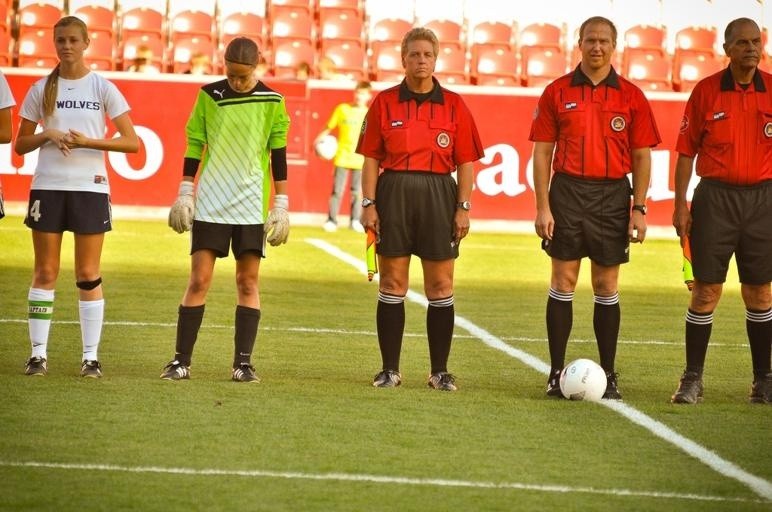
[168,181,195,235]
[263,194,290,247]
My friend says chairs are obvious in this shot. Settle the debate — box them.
[0,0,772,94]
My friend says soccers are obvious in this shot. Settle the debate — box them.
[313,135,338,161]
[560,359,608,402]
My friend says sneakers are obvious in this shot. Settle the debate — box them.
[232,362,261,383]
[670,370,704,403]
[372,368,401,388]
[349,220,365,232]
[81,359,102,379]
[428,371,457,391]
[603,368,621,399]
[159,359,191,381]
[748,368,772,403]
[25,356,49,376]
[324,220,337,232]
[546,367,563,395]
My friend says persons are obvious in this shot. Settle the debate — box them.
[312,80,374,234]
[0,68,17,220]
[158,38,291,382]
[528,15,664,400]
[670,16,771,402]
[353,26,486,390]
[15,14,140,378]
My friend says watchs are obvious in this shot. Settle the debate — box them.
[631,204,648,214]
[457,200,472,211]
[360,198,376,207]
